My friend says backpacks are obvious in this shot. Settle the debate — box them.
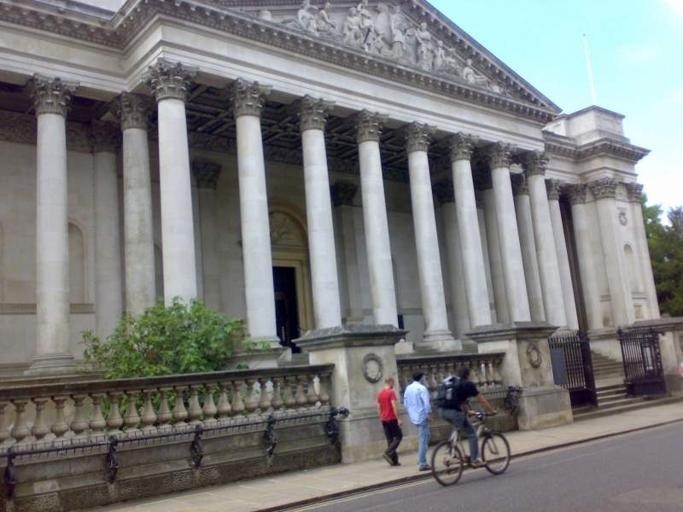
[432,375,462,411]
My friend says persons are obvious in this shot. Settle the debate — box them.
[404,371,433,471]
[280,1,489,90]
[375,376,404,465]
[437,366,497,468]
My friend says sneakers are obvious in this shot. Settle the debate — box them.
[417,464,435,471]
[381,452,395,466]
[390,462,401,467]
[470,459,487,468]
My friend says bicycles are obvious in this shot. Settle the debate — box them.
[430,409,510,486]
